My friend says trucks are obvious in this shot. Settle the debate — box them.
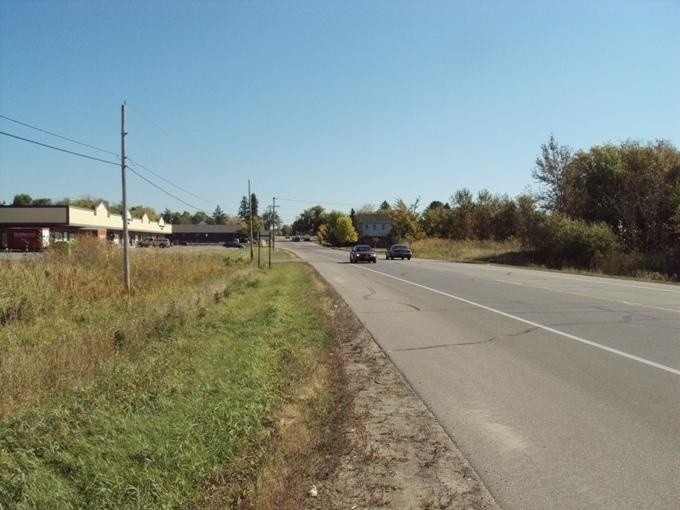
[140,237,170,248]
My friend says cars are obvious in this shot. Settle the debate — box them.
[385,244,412,260]
[285,233,312,242]
[349,245,377,263]
[223,242,246,248]
